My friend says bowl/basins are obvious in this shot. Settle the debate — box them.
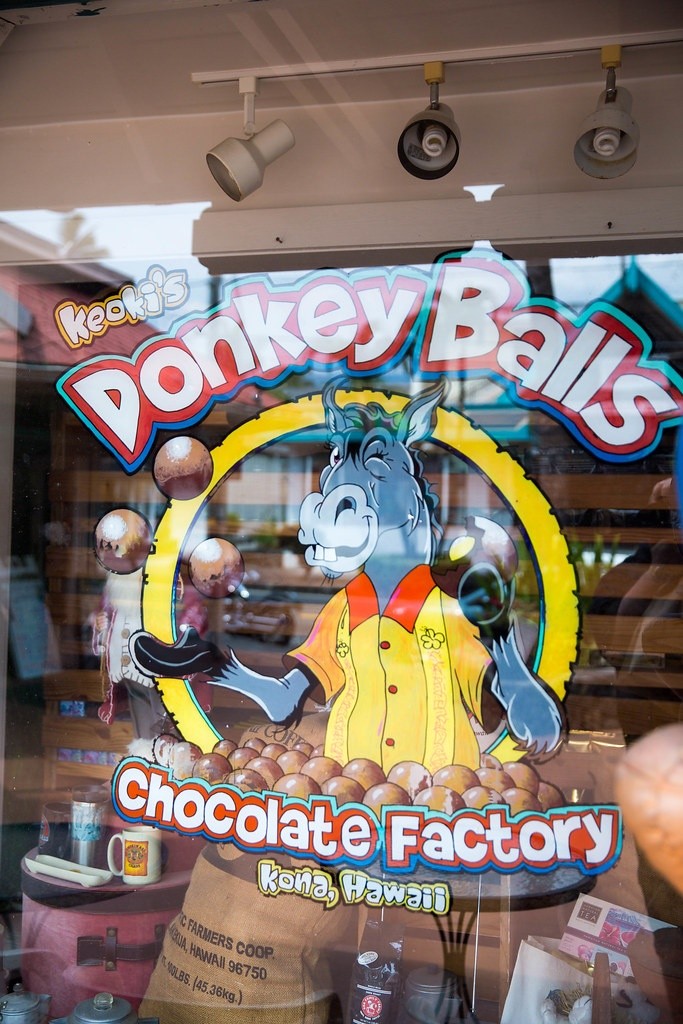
[627,928,683,1018]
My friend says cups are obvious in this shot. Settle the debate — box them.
[71,785,110,867]
[38,802,70,859]
[107,826,162,885]
